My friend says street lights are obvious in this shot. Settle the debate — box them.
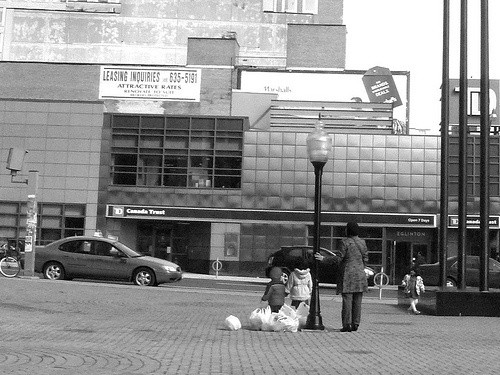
[299,113,341,335]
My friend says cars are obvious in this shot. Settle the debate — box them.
[265,245,376,287]
[418,255,500,289]
[20,235,185,287]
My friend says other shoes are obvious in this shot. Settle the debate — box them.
[414,311,421,315]
[406,310,410,315]
[352,324,359,331]
[339,324,352,332]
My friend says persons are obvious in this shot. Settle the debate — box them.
[261,267,285,313]
[411,251,425,276]
[402,269,425,315]
[314,222,369,332]
[285,256,313,332]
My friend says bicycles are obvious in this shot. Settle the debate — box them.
[0,243,21,280]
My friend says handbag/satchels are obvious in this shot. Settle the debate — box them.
[224,316,242,332]
[249,301,311,332]
